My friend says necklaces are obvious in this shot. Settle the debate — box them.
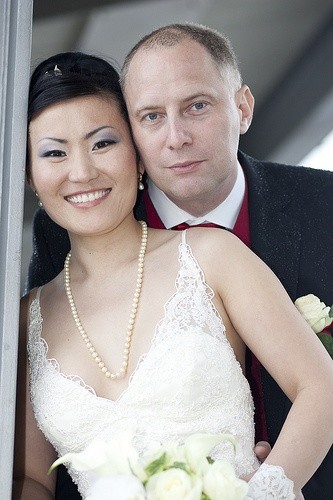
[64,219,148,378]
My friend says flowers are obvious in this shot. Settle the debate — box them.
[294,293,333,360]
[48,433,249,500]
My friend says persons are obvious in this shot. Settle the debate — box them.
[11,51,333,500]
[28,22,333,500]
